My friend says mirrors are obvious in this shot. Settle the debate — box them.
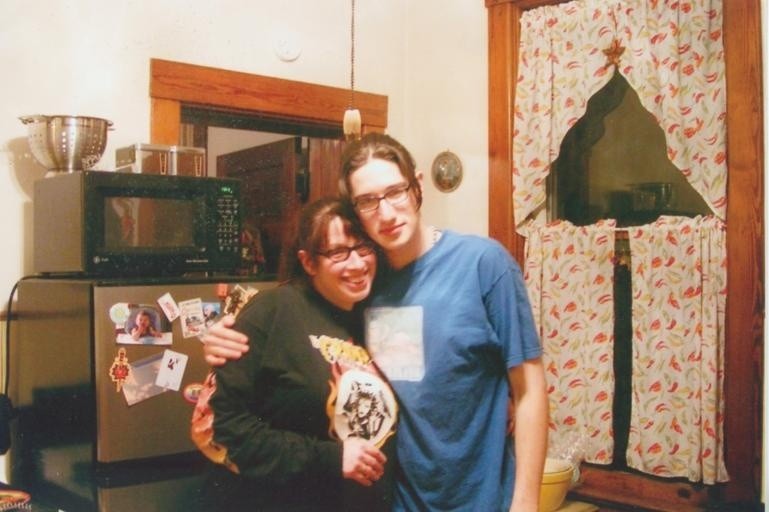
[149,57,390,279]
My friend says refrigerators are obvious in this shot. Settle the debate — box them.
[6,279,286,512]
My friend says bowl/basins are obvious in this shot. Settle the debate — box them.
[537,457,575,512]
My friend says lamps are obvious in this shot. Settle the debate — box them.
[343,0,362,140]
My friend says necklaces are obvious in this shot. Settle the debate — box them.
[428,225,438,244]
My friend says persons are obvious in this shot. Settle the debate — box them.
[184,194,516,512]
[126,308,164,342]
[196,130,550,511]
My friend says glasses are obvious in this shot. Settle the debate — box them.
[354,178,418,212]
[317,239,375,261]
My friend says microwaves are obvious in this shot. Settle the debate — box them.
[31,170,243,280]
